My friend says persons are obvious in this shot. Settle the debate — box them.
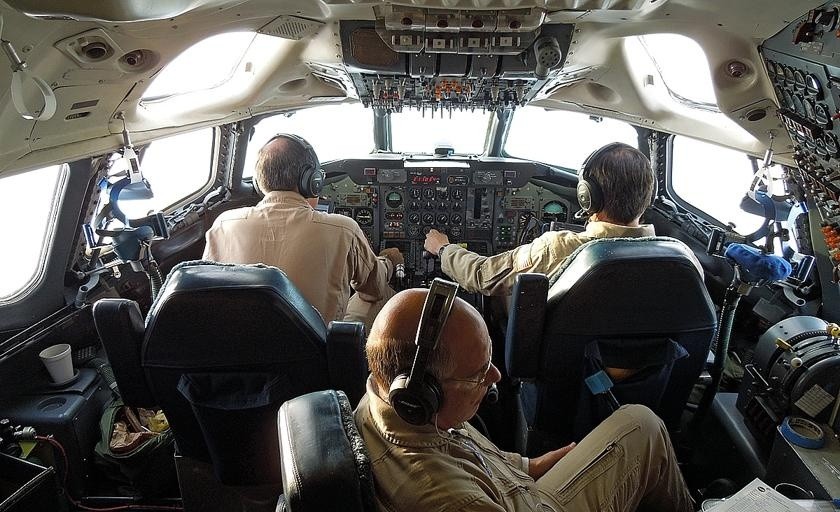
[202,136,406,338]
[421,144,660,315]
[349,288,698,512]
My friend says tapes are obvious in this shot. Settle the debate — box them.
[782,415,824,449]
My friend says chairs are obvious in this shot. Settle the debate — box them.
[504,238,716,461]
[275,389,369,511]
[93,261,366,512]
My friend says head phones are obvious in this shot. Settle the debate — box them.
[575,141,659,217]
[251,131,326,200]
[386,274,460,425]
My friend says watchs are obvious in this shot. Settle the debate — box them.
[438,243,450,258]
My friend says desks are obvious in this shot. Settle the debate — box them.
[712,394,773,483]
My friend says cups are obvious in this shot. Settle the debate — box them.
[39,342,75,386]
